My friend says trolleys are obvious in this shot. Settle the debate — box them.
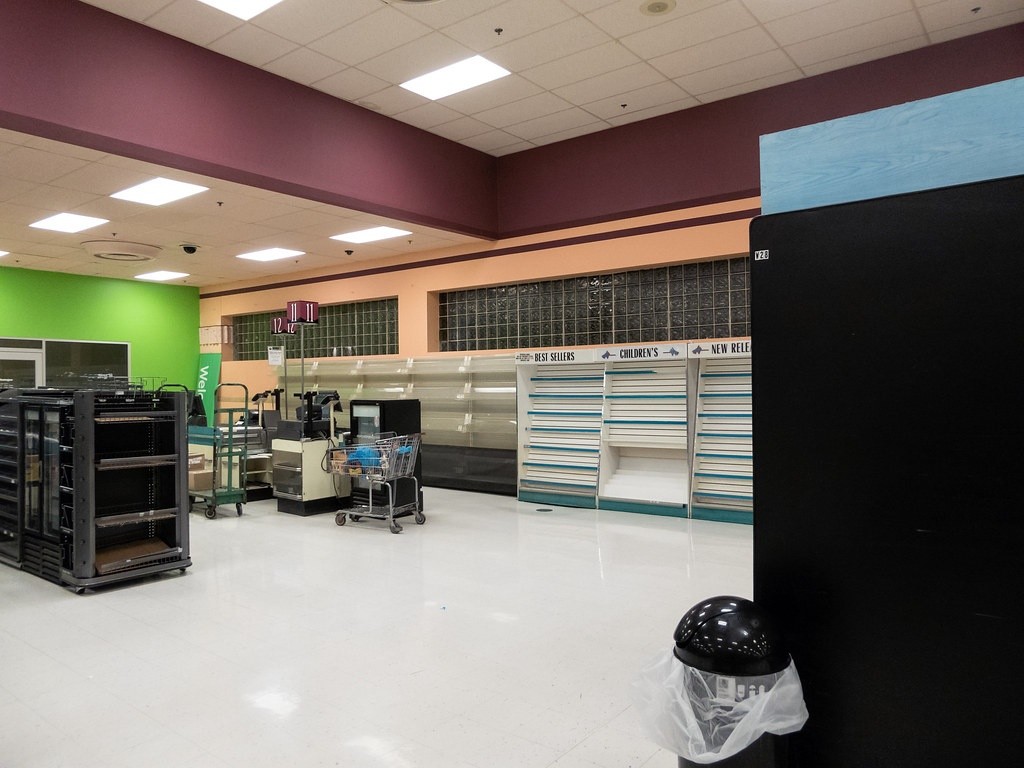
[154,379,249,520]
[323,430,426,534]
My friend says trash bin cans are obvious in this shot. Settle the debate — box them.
[672,595,791,768]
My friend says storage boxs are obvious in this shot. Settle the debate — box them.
[189,470,219,491]
[188,452,205,473]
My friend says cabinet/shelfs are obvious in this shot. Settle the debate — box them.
[0,380,194,594]
[278,340,753,525]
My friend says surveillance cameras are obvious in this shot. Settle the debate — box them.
[345,251,353,255]
[184,245,197,254]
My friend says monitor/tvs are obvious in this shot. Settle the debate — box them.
[194,394,208,426]
[319,390,343,412]
